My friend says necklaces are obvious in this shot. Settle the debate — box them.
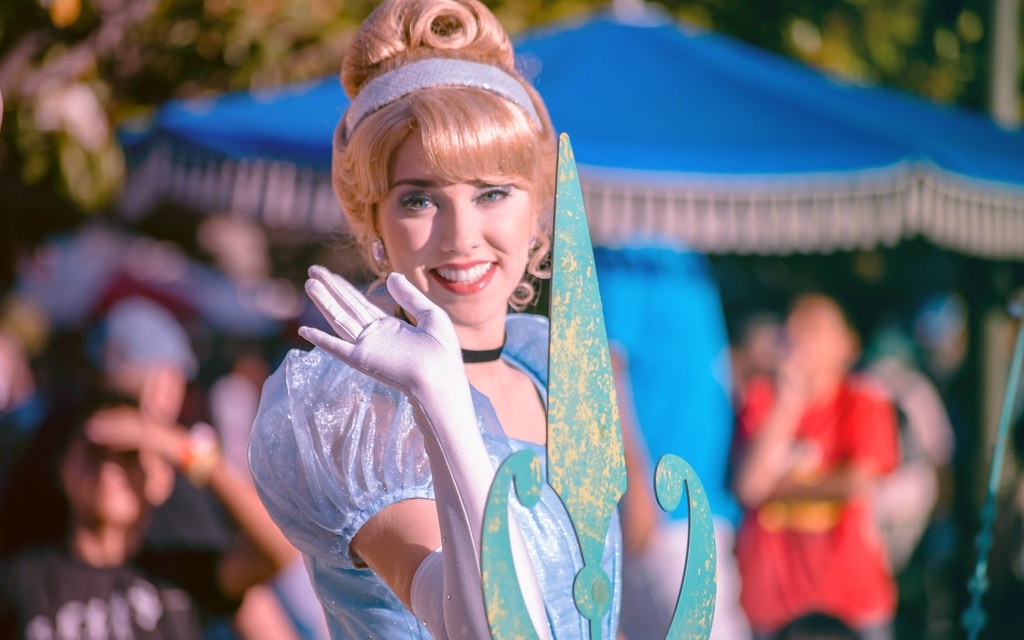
[396,306,507,366]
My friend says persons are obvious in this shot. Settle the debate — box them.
[245,2,650,639]
[0,212,1022,640]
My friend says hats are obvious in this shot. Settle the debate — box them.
[104,296,197,379]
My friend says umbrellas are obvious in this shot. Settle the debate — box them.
[118,0,1024,265]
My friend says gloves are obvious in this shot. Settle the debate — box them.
[298,265,552,640]
